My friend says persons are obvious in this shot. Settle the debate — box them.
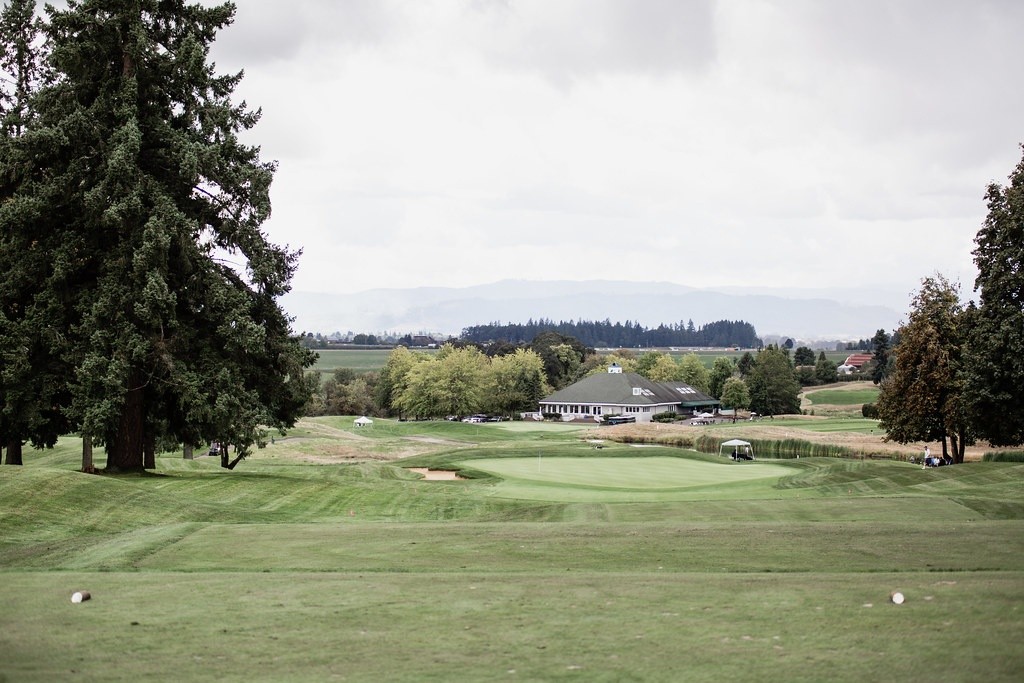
[922,446,948,470]
[744,446,748,456]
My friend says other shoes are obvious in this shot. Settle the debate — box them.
[921,467,925,469]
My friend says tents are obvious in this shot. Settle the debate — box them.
[720,438,755,460]
[354,416,373,427]
[698,412,713,419]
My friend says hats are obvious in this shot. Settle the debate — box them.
[924,446,927,448]
